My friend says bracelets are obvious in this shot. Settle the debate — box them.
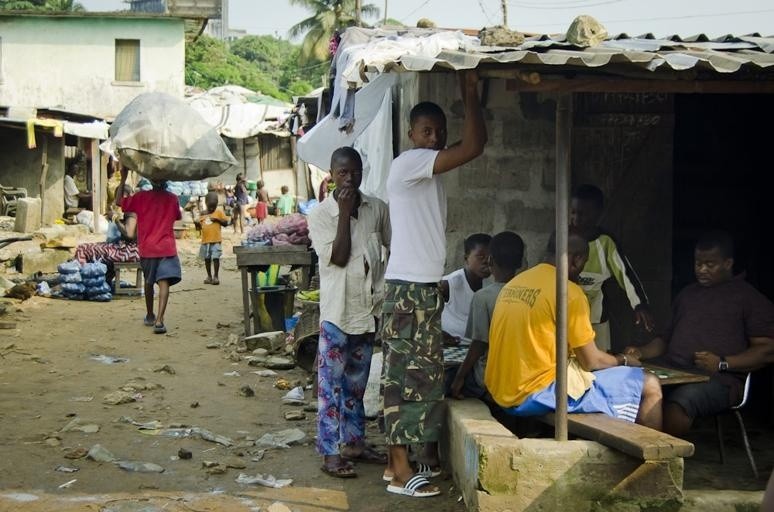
[112,214,119,224]
[617,352,628,366]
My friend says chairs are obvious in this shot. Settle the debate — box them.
[695,367,759,479]
[0,184,27,217]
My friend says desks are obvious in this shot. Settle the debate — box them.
[627,360,710,386]
[233,244,319,337]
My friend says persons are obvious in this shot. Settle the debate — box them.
[74,185,140,293]
[210,179,232,209]
[107,163,153,223]
[103,179,182,334]
[232,172,251,234]
[541,185,658,353]
[448,231,524,401]
[275,185,297,217]
[195,191,229,285]
[189,195,207,236]
[255,180,273,225]
[439,233,492,398]
[622,235,773,439]
[64,163,92,225]
[484,229,665,433]
[377,68,488,498]
[319,176,332,202]
[306,146,392,478]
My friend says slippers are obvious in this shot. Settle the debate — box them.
[341,447,388,465]
[386,474,441,498]
[320,462,357,478]
[144,315,156,325]
[154,322,166,332]
[383,463,441,482]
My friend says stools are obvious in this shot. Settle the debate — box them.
[112,262,142,295]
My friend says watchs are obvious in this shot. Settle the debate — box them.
[719,355,729,374]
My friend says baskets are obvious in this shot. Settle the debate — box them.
[290,309,320,373]
